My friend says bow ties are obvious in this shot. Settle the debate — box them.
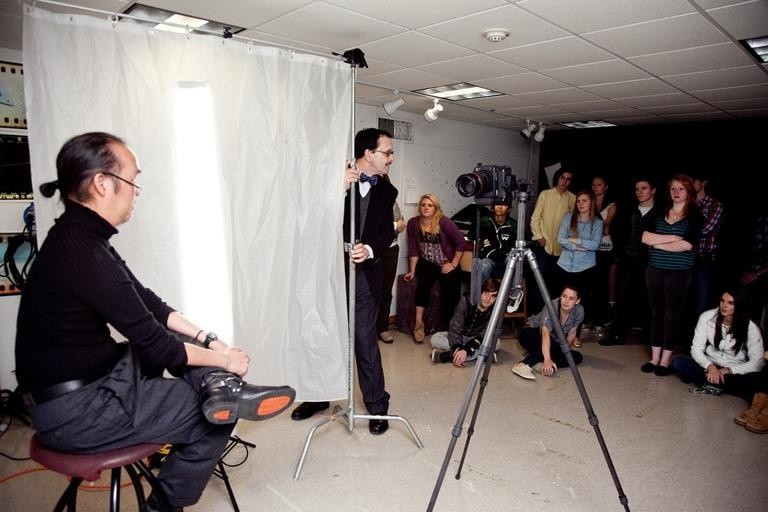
[359,172,377,186]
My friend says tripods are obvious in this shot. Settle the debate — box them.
[426,210,633,512]
[291,62,425,483]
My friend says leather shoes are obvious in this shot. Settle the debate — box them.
[201,370,296,425]
[369,411,388,435]
[291,402,329,420]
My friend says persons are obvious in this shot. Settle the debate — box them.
[13,130,297,512]
[402,163,768,434]
[376,173,408,343]
[291,127,399,435]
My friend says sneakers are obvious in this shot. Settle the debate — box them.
[534,363,557,376]
[641,362,659,373]
[432,349,451,363]
[655,365,671,376]
[573,341,582,348]
[702,386,722,396]
[511,363,536,380]
[688,385,708,394]
[378,332,394,344]
[484,352,498,363]
[414,322,425,341]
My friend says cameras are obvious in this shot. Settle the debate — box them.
[454,165,515,208]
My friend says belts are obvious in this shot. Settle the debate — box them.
[12,344,126,409]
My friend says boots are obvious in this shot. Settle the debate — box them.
[745,405,768,434]
[733,392,768,426]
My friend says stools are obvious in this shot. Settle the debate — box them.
[397,274,442,337]
[29,434,163,512]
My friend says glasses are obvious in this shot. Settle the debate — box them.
[371,150,394,157]
[102,172,143,197]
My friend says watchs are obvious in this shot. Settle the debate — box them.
[203,332,218,348]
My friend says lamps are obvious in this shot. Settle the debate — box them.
[356,81,552,140]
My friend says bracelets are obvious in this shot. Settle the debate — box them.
[194,329,204,340]
